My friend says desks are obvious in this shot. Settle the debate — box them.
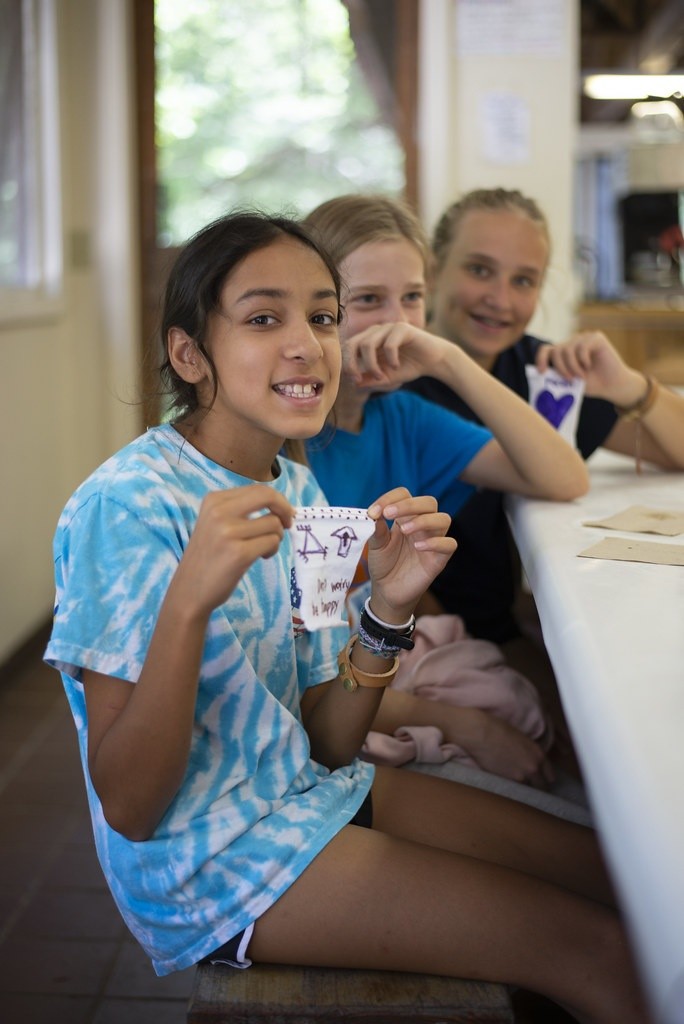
[509,385,684,1024]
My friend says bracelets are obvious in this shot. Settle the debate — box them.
[357,598,414,659]
[338,634,399,694]
[618,373,658,474]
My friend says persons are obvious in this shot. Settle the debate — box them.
[42,210,648,1024]
[415,188,684,695]
[282,198,594,827]
